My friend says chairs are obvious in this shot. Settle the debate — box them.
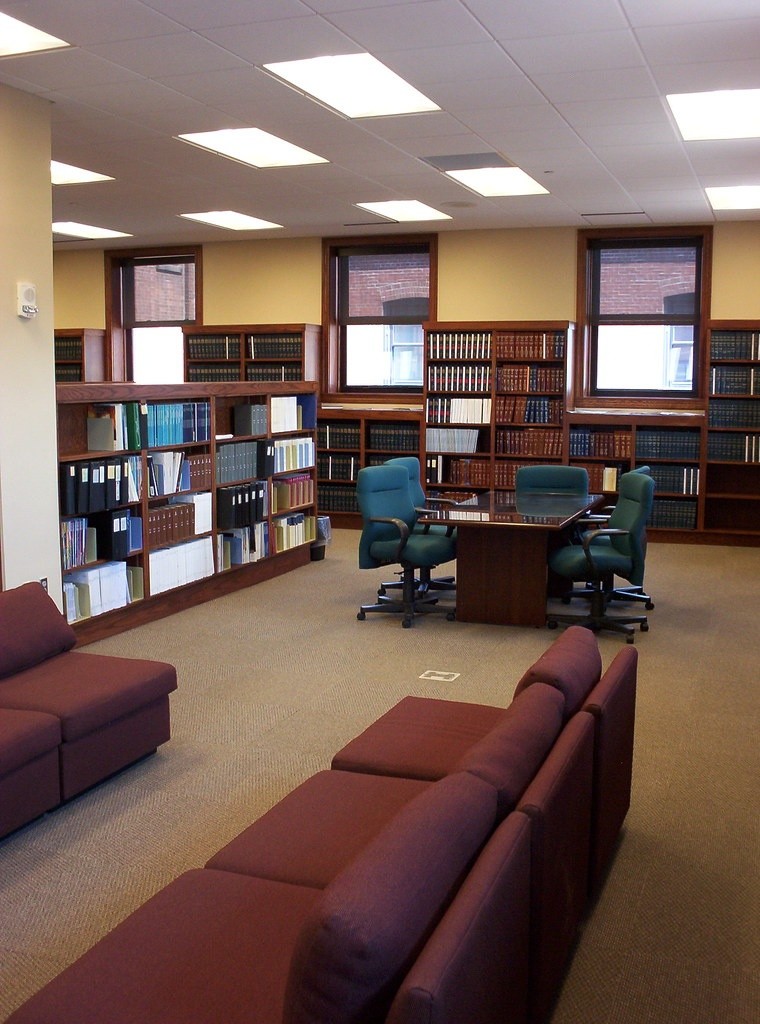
[549,466,656,643]
[357,458,457,628]
[515,465,588,490]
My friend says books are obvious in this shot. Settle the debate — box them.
[86,395,305,451]
[185,336,303,381]
[60,435,315,622]
[316,419,420,512]
[570,429,699,529]
[708,330,760,462]
[425,331,563,525]
[53,337,81,381]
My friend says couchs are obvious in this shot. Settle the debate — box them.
[0,651,178,842]
[0,627,638,1024]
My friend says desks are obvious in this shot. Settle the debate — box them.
[418,490,603,633]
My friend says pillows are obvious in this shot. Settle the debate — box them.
[0,582,77,670]
[281,629,600,1024]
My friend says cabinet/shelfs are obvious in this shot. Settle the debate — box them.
[54,328,106,383]
[56,381,317,651]
[182,319,760,547]
